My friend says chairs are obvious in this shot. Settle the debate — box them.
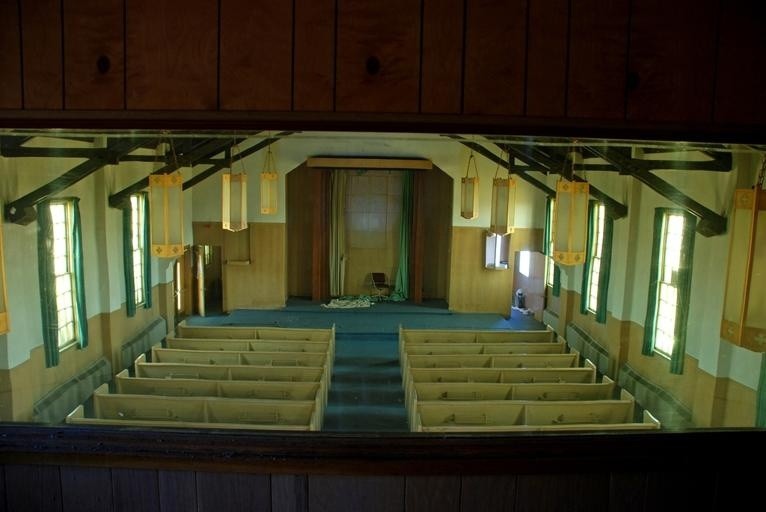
[370,271,391,302]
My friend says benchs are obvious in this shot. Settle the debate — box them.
[61,321,336,429]
[393,323,659,432]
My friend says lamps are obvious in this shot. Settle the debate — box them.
[554,138,589,266]
[458,145,480,221]
[258,128,278,216]
[491,135,514,236]
[221,128,247,234]
[147,130,182,259]
